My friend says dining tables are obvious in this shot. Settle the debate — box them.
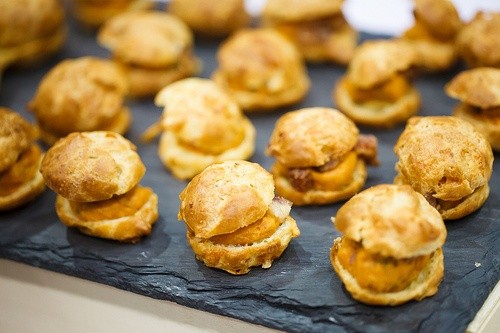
[1,0,500,333]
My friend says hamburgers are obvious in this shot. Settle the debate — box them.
[39,128,162,246]
[261,1,359,65]
[333,38,423,127]
[97,9,204,98]
[0,106,51,209]
[445,65,500,151]
[393,114,494,221]
[28,53,133,146]
[404,1,463,72]
[0,0,72,69]
[329,183,446,307]
[457,10,500,65]
[179,159,300,274]
[213,27,311,114]
[265,107,380,206]
[156,75,258,179]
[167,1,253,42]
